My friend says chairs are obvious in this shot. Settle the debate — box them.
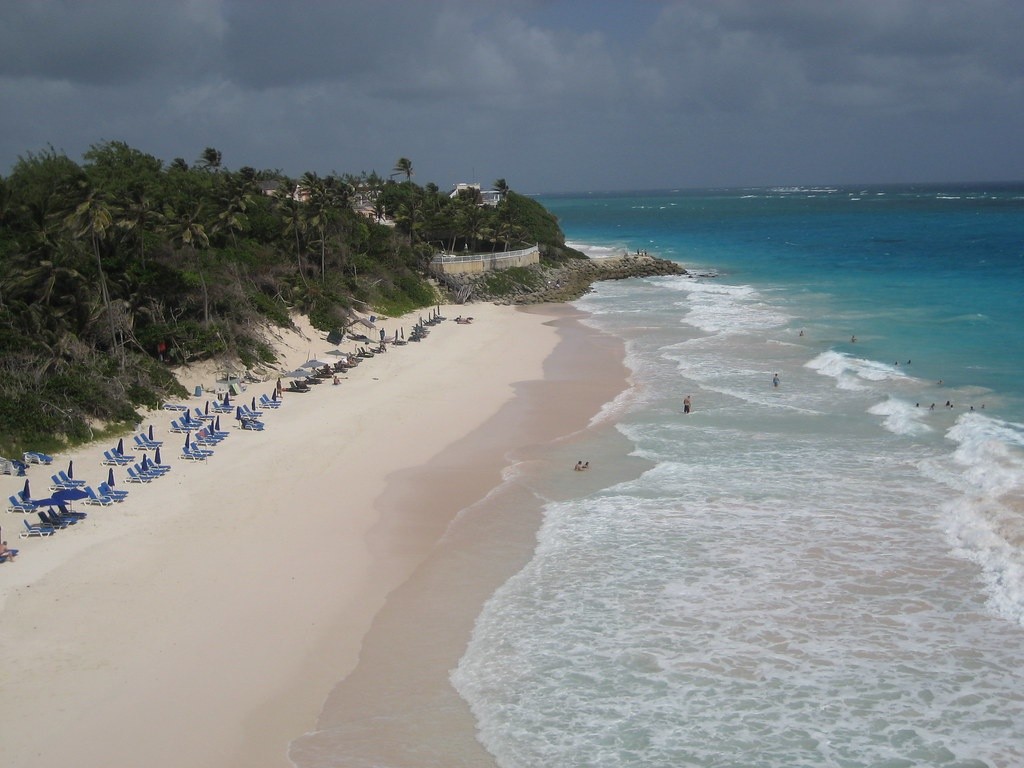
[290,315,448,393]
[0,385,282,564]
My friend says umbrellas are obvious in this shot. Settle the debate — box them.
[23,304,441,500]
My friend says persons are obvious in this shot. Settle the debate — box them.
[683,396,691,413]
[916,403,919,407]
[981,405,984,408]
[931,403,935,410]
[575,461,589,471]
[0,541,15,563]
[970,406,974,410]
[800,331,804,336]
[454,315,461,323]
[636,248,647,255]
[938,380,945,383]
[334,376,340,383]
[851,335,856,342]
[895,362,898,365]
[946,401,953,408]
[907,360,911,364]
[277,378,283,398]
[773,374,780,387]
